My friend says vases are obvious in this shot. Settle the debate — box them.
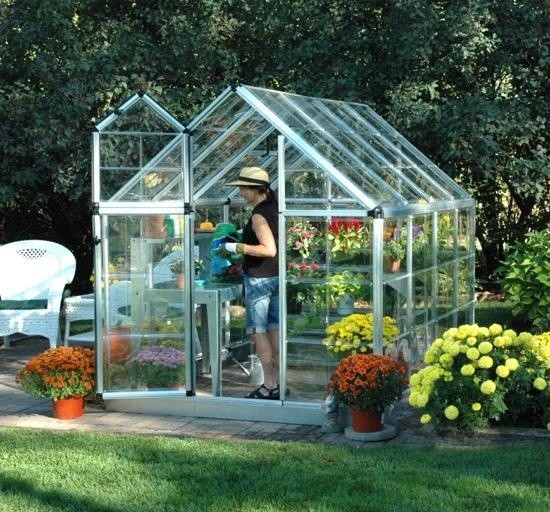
[51,396,84,420]
[384,256,401,274]
[147,383,181,391]
[349,407,383,433]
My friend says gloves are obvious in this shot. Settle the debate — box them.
[219,242,247,259]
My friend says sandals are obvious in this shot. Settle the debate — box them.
[284,390,290,396]
[245,384,280,400]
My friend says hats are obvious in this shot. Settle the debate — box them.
[224,165,271,188]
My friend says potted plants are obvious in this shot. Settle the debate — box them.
[324,271,369,316]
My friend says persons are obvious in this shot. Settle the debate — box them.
[215,167,291,399]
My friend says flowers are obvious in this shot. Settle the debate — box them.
[383,214,451,257]
[328,353,410,413]
[16,346,95,400]
[123,345,186,386]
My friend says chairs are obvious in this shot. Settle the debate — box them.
[0,240,76,349]
[63,281,131,350]
